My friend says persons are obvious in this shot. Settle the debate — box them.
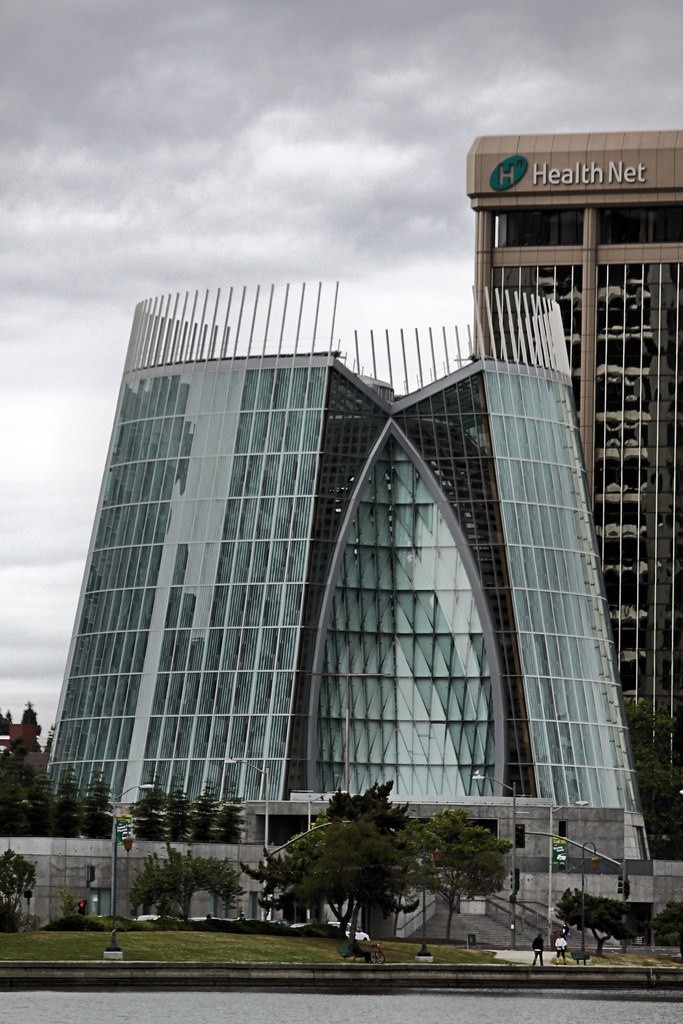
[531,933,545,966]
[553,923,571,965]
[351,940,370,964]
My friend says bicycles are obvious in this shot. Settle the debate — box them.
[370,942,386,964]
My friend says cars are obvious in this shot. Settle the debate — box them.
[326,920,372,942]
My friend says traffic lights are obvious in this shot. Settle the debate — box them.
[223,755,272,921]
[86,863,96,884]
[510,867,521,892]
[617,874,628,895]
[623,879,630,899]
[77,899,87,916]
[23,890,33,898]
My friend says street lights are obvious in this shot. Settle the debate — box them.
[546,800,592,949]
[107,782,157,951]
[471,770,518,949]
[305,789,348,924]
[305,671,393,789]
